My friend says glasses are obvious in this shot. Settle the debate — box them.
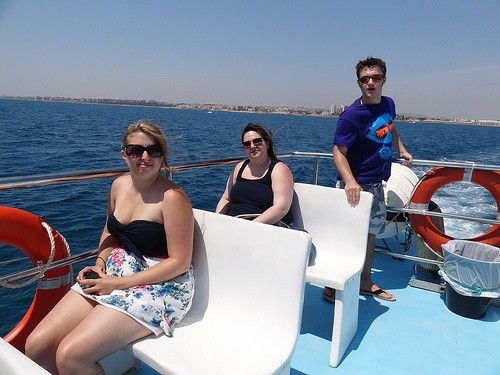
[359,74,384,83]
[242,138,263,149]
[125,144,164,158]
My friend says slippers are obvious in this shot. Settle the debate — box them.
[323,287,335,303]
[360,288,396,301]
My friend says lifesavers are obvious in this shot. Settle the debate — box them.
[408,161,500,253]
[0,206,73,355]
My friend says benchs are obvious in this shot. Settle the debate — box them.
[122,208,312,375]
[292,182,374,368]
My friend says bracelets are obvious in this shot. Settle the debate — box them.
[95,256,106,274]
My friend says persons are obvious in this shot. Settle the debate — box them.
[323,56,413,301]
[24,121,195,375]
[215,123,294,227]
